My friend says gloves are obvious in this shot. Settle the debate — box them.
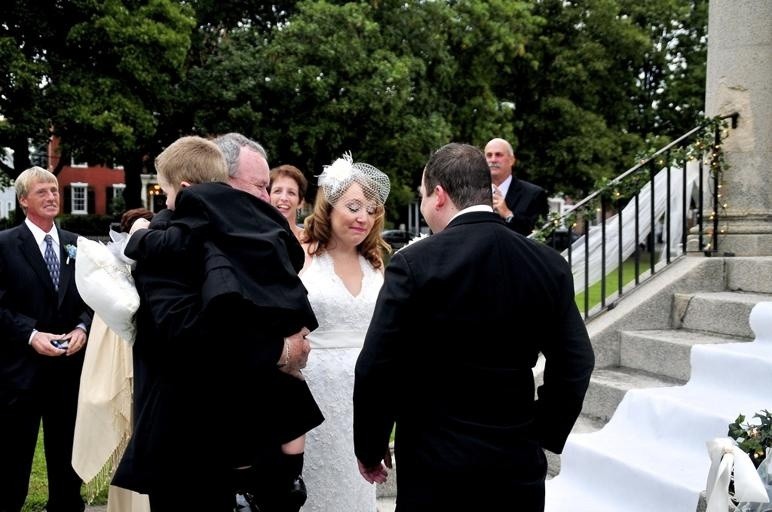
[541,447,561,480]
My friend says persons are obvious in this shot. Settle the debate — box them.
[267,164,310,243]
[0,166,85,512]
[353,143,594,512]
[483,138,546,237]
[69,207,156,511]
[301,149,394,511]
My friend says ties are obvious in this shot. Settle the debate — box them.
[44,235,60,291]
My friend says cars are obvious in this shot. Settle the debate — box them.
[382,230,415,248]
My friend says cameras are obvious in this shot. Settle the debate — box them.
[51,339,69,349]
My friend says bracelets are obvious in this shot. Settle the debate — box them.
[284,337,291,367]
[505,216,513,223]
